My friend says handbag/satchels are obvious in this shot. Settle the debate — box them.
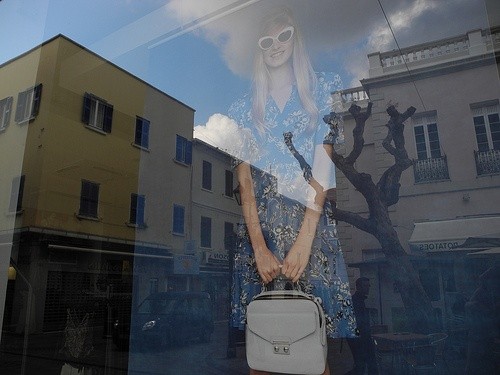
[242,275,328,375]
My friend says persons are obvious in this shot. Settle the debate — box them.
[223,12,364,374]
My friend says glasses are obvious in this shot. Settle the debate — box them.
[256,25,293,50]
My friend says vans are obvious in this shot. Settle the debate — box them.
[112,288,216,348]
